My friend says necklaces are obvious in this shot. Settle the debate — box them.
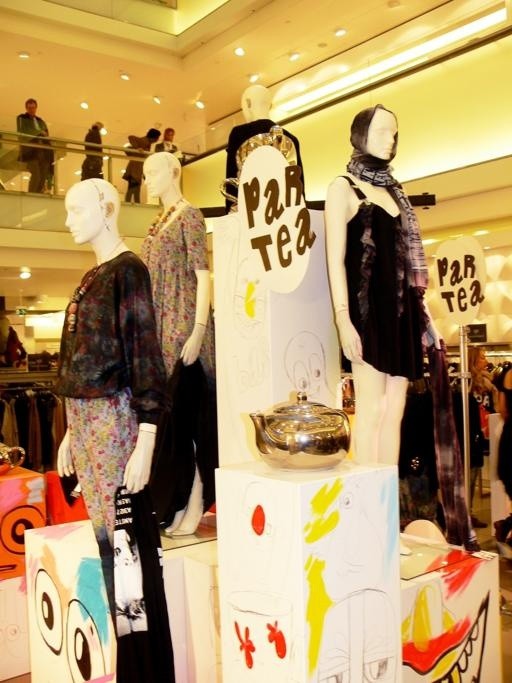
[143,194,186,264]
[68,235,126,331]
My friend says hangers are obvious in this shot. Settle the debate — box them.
[0,383,63,403]
[422,351,512,392]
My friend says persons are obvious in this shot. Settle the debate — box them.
[54,177,174,683]
[130,149,214,541]
[15,98,49,193]
[450,344,511,569]
[154,128,179,153]
[123,127,159,202]
[42,132,54,192]
[327,103,426,467]
[81,120,104,182]
[223,86,304,214]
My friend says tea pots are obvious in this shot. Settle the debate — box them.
[1,441,27,476]
[248,394,353,470]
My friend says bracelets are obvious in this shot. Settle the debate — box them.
[332,301,349,312]
[194,320,208,328]
[137,427,159,435]
